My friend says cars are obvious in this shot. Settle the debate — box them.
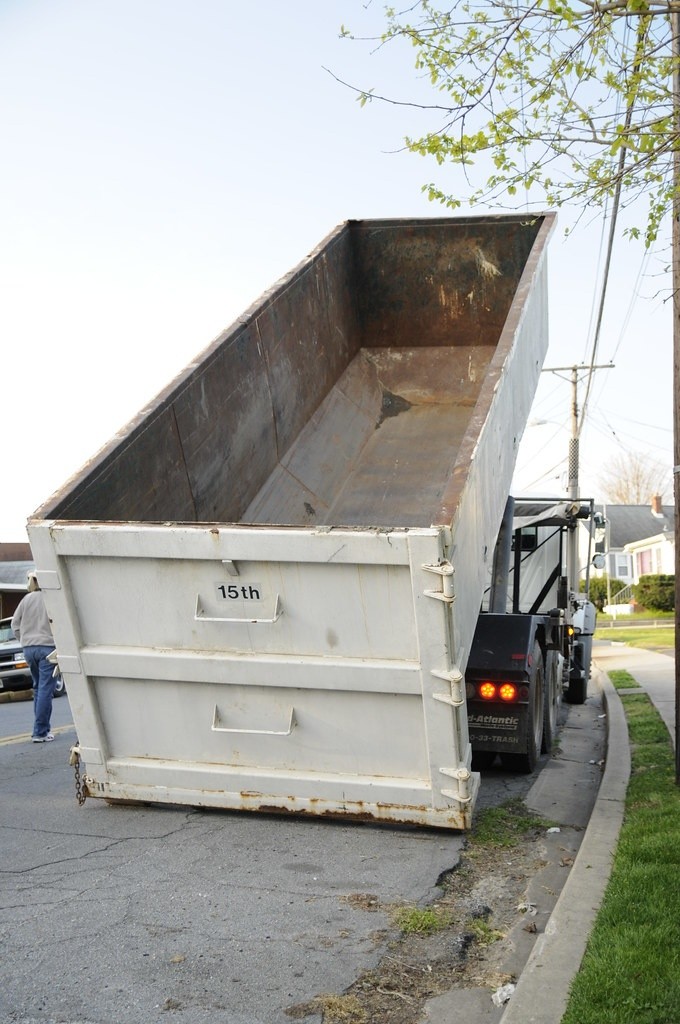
[0,639,65,698]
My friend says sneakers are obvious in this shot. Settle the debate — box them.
[32,733,55,742]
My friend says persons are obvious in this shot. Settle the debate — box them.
[10,571,60,742]
